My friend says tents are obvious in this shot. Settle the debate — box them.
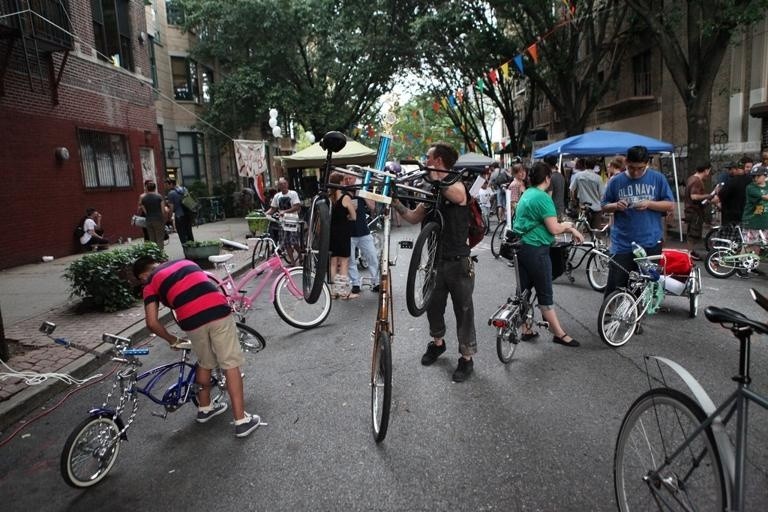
[455,152,497,166]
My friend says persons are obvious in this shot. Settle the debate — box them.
[139,178,194,246]
[477,145,768,348]
[131,255,261,439]
[240,184,277,213]
[80,208,103,253]
[265,171,379,301]
[391,145,477,383]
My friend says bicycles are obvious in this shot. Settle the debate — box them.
[37,322,227,489]
[612,287,767,512]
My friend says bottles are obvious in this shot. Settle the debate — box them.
[630,241,656,273]
[758,229,766,246]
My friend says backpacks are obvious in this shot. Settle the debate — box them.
[171,186,202,220]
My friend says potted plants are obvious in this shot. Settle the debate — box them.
[179,239,225,269]
[60,239,170,314]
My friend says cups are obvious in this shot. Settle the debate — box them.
[561,222,574,243]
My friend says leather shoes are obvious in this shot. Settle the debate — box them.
[553,334,580,347]
[522,331,539,341]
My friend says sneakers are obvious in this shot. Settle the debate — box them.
[235,414,260,438]
[691,250,700,259]
[748,268,760,276]
[197,401,228,423]
[421,339,446,366]
[452,357,473,382]
[736,271,749,279]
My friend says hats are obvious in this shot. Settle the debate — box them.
[749,167,765,176]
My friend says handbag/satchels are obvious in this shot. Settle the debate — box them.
[499,230,522,261]
[468,194,487,249]
[75,224,84,239]
[131,214,147,228]
